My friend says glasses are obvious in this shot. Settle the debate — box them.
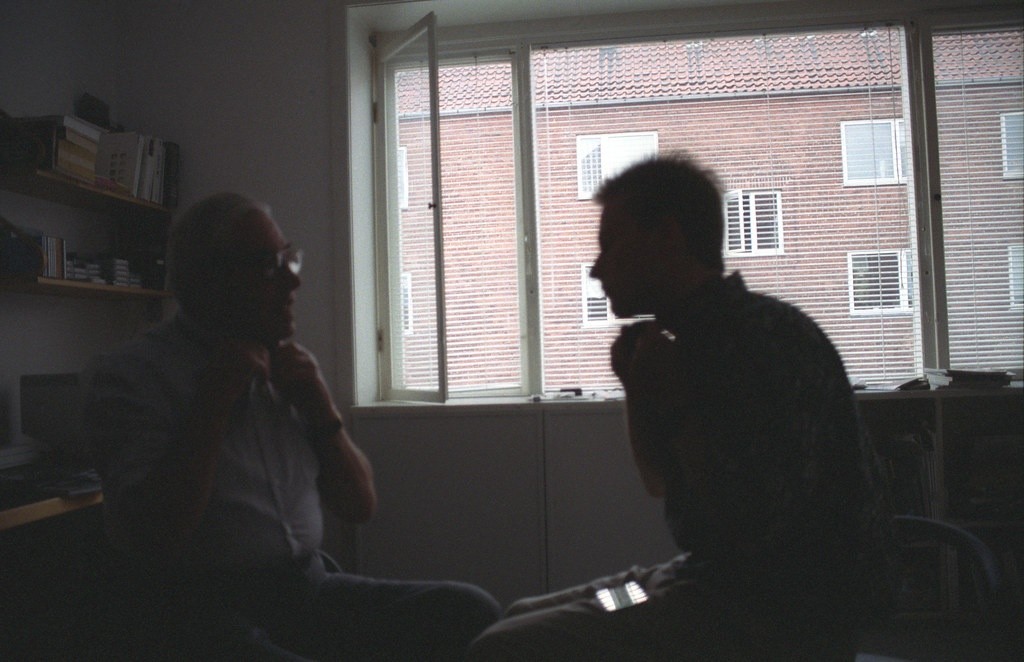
[250,246,302,284]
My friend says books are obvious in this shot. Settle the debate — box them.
[30,111,166,289]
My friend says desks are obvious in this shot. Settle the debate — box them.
[0,453,110,662]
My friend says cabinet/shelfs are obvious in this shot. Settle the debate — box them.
[0,159,184,301]
[852,386,1024,622]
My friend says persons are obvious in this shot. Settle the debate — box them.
[83,190,502,662]
[462,155,906,662]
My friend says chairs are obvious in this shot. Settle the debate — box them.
[825,516,1010,662]
